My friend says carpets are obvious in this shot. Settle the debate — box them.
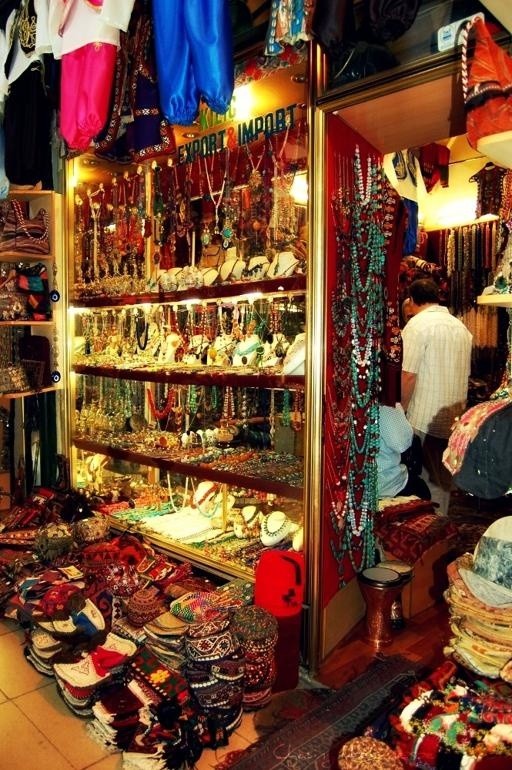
[214,653,435,770]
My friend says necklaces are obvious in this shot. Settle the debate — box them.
[327,145,386,589]
[72,125,311,589]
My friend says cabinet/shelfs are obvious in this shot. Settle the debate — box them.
[0,190,66,398]
[64,40,312,604]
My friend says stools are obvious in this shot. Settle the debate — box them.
[377,560,414,628]
[359,568,400,647]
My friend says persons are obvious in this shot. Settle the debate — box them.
[375,278,473,500]
